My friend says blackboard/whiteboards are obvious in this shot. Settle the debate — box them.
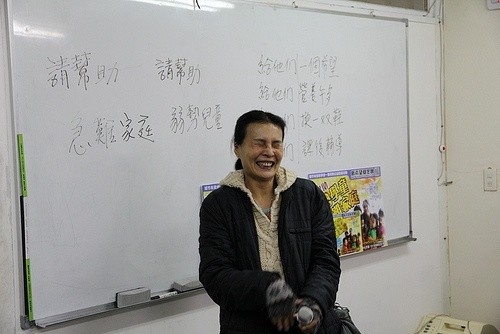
[3,0,415,334]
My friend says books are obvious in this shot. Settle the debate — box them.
[200,182,221,203]
[309,166,388,257]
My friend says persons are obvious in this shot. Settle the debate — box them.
[199,110,342,334]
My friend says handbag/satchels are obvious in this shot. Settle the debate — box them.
[313,303,361,334]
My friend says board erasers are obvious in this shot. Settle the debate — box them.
[110,285,154,308]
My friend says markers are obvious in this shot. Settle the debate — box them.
[147,289,181,300]
